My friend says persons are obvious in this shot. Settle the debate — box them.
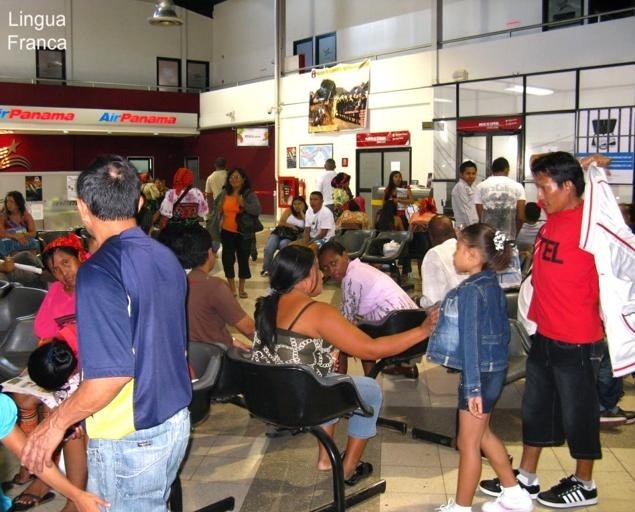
[479,151,635,508]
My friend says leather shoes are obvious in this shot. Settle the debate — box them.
[383,363,419,377]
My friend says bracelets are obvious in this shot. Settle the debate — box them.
[578,157,584,165]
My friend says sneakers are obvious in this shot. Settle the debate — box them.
[252,249,257,260]
[599,405,634,428]
[435,469,599,512]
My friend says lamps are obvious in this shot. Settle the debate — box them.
[144,1,186,27]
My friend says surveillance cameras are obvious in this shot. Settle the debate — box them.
[267,105,273,114]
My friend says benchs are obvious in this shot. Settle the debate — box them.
[0,212,541,509]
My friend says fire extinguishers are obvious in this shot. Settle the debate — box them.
[282,181,290,202]
[298,178,305,197]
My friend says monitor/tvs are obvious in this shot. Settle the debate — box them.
[236,127,269,148]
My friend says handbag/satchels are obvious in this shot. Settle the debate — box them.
[235,211,264,233]
[271,225,299,241]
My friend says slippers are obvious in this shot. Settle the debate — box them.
[12,491,55,509]
[1,472,35,491]
[345,462,374,486]
